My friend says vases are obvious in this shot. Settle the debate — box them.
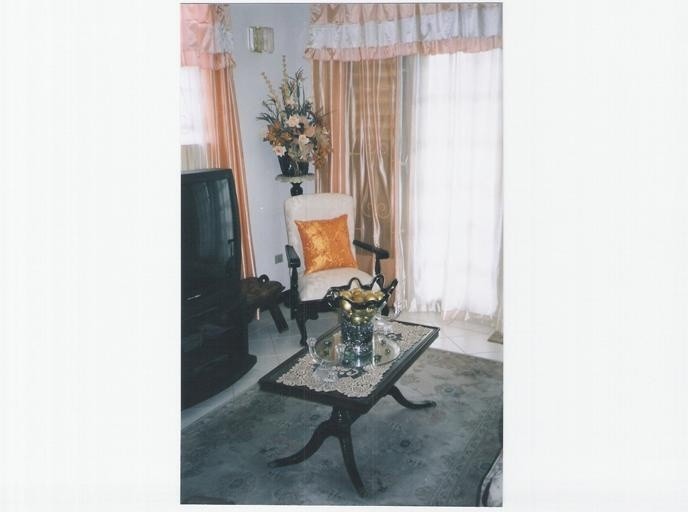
[278,152,309,176]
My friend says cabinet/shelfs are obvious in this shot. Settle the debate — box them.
[181,276,285,411]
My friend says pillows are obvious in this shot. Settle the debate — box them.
[294,214,357,277]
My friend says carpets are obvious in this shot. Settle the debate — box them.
[181,344,503,506]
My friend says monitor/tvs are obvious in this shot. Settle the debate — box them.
[181,169,242,322]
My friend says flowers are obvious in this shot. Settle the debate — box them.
[256,55,336,170]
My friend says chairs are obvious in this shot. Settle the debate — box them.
[284,192,390,346]
